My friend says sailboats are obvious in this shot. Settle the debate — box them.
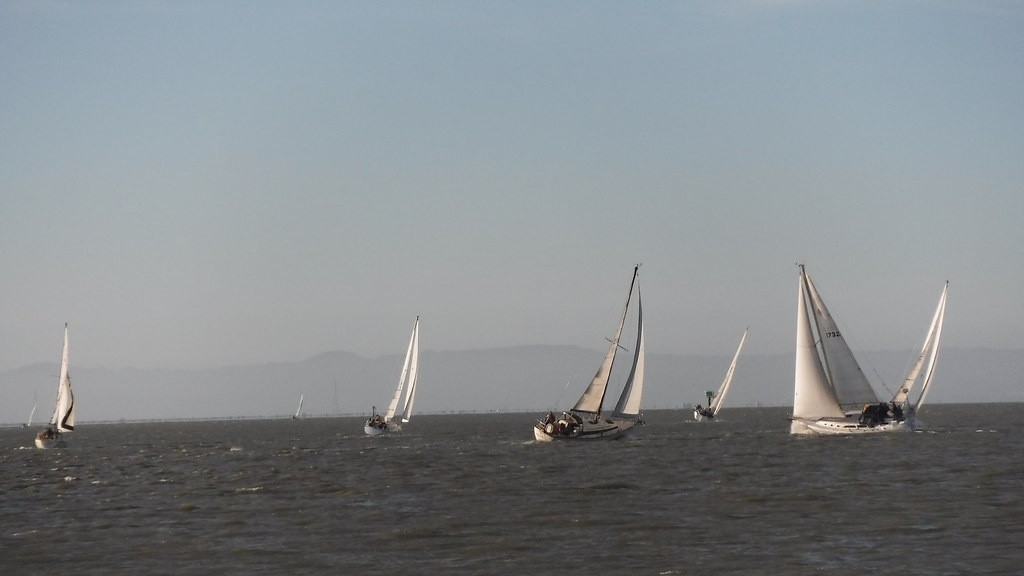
[691,329,749,422]
[788,264,948,435]
[292,394,303,422]
[35,322,75,448]
[21,405,36,429]
[364,314,420,435]
[533,264,645,444]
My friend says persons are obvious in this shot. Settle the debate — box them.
[37,424,59,441]
[366,413,389,435]
[857,399,903,428]
[695,404,718,419]
[537,409,583,438]
